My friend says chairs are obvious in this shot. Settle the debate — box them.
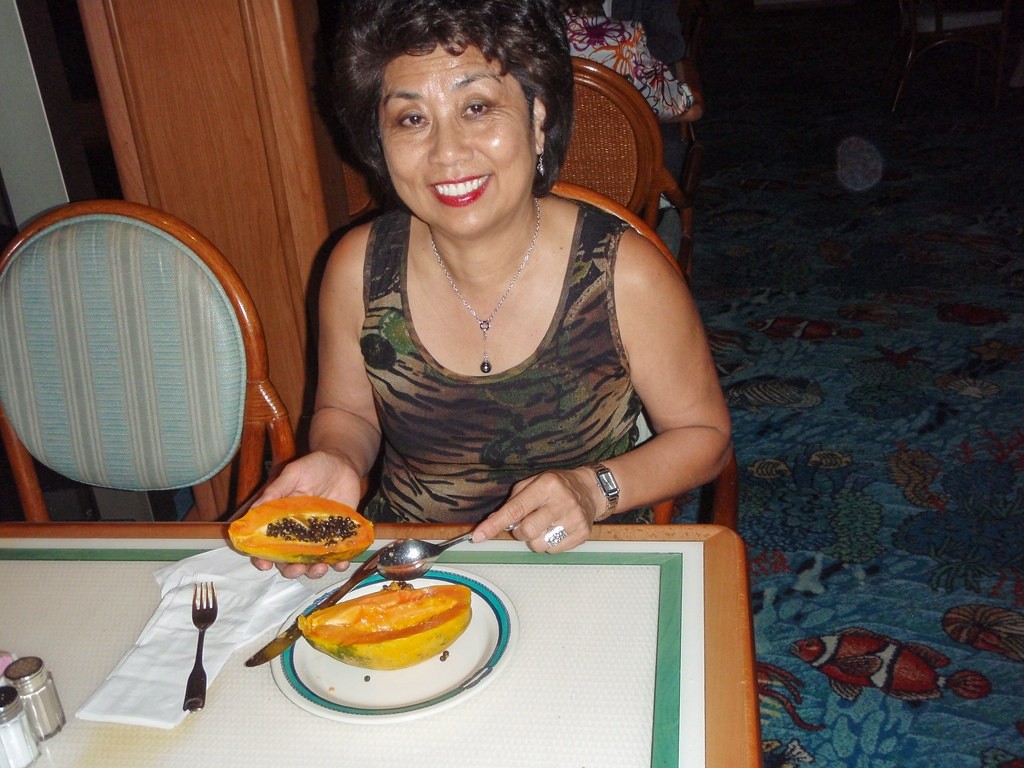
[556,0,739,529]
[0,201,296,525]
[879,0,1011,113]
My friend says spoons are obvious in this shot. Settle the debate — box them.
[376,532,472,581]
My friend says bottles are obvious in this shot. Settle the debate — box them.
[0,687,40,767]
[3,656,65,740]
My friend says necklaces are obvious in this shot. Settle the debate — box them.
[429,197,541,373]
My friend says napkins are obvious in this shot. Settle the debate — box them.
[77,545,316,729]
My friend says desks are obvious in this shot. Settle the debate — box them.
[0,519,763,768]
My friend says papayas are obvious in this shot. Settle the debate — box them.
[297,584,472,669]
[228,496,374,565]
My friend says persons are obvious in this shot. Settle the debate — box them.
[546,0,706,259]
[246,1,734,578]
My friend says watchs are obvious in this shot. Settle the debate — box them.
[576,461,619,522]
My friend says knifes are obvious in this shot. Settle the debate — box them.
[245,551,382,668]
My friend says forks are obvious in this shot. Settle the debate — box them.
[183,581,218,712]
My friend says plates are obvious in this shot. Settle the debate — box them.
[270,565,519,724]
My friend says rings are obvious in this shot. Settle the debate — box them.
[544,525,569,548]
